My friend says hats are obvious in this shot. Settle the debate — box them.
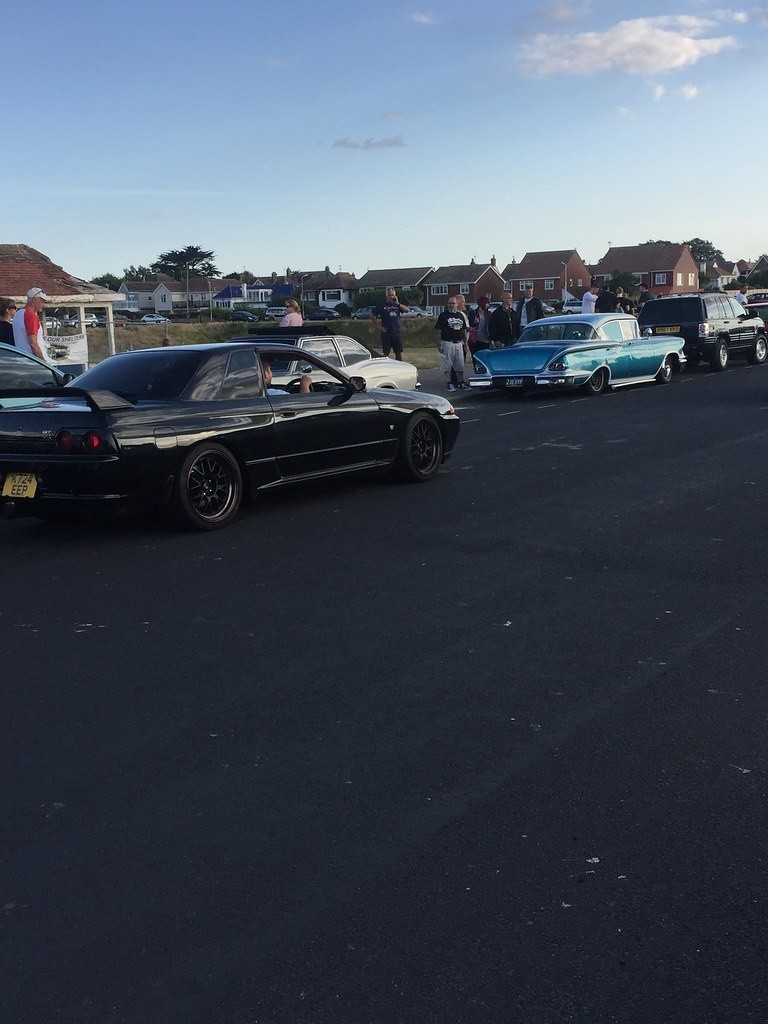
[26,287,51,303]
[638,282,648,289]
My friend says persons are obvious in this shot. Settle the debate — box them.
[261,359,312,394]
[370,288,422,386]
[0,298,16,346]
[12,286,55,386]
[434,283,663,392]
[280,299,303,327]
[736,284,750,305]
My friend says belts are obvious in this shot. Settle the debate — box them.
[452,340,462,343]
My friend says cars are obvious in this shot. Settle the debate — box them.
[302,309,341,321]
[40,317,61,330]
[541,303,555,315]
[0,341,461,532]
[351,306,382,321]
[737,292,768,332]
[231,310,260,322]
[96,313,129,329]
[140,313,171,326]
[464,301,524,315]
[399,306,434,319]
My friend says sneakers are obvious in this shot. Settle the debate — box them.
[446,384,456,392]
[457,384,472,391]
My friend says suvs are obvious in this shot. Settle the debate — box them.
[63,313,99,329]
[635,291,768,371]
[562,300,596,315]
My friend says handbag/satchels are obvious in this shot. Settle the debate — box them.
[468,330,477,345]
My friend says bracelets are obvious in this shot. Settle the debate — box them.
[397,303,400,307]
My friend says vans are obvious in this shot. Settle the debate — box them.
[264,306,287,323]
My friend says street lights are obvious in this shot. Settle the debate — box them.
[301,274,309,319]
[208,282,212,322]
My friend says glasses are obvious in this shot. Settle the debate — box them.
[286,305,292,308]
[9,306,16,310]
[33,289,45,298]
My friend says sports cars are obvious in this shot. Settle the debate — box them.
[468,312,687,400]
[220,332,421,392]
